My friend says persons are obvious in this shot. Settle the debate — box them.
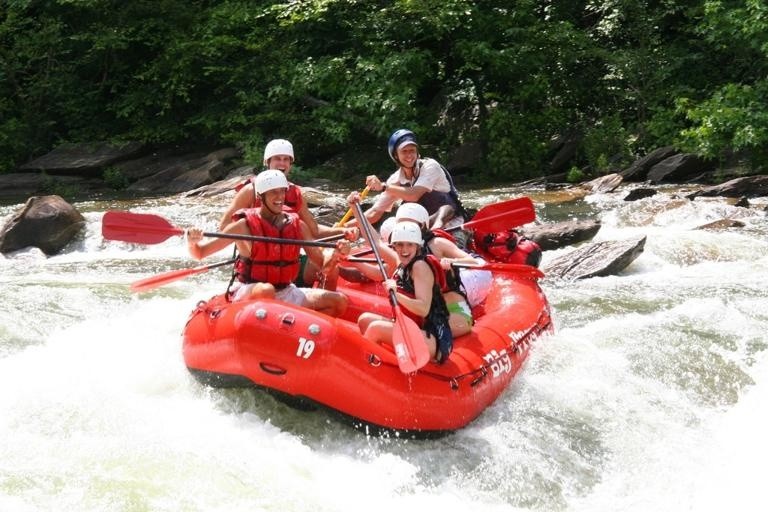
[330,128,470,250]
[184,167,351,317]
[336,190,480,365]
[217,137,362,287]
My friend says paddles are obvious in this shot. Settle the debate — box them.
[103,211,339,248]
[352,198,535,258]
[354,199,430,373]
[131,234,345,291]
[348,257,544,277]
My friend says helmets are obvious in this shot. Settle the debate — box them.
[263,139,295,167]
[379,216,397,242]
[254,169,289,197]
[396,202,431,230]
[390,221,426,247]
[388,129,416,163]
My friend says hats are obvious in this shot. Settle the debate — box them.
[395,135,418,150]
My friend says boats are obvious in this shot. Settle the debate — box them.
[176,238,555,441]
